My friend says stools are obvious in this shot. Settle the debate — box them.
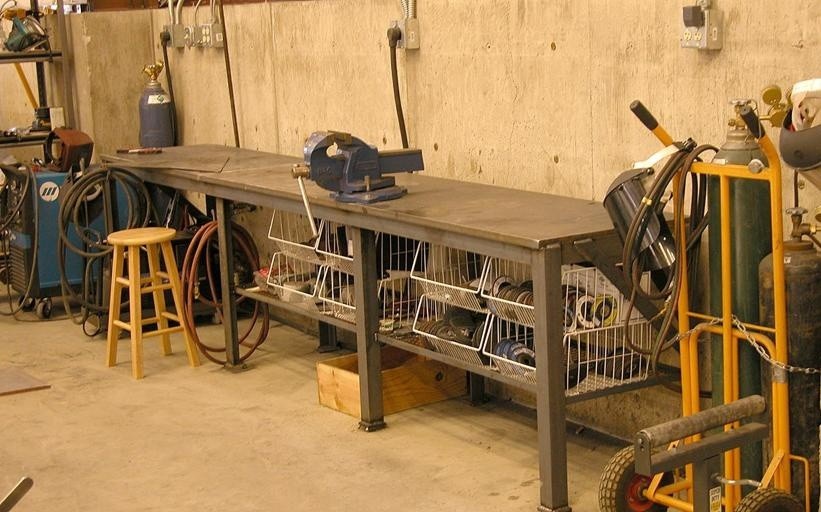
[102,222,203,380]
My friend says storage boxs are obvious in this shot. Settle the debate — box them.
[316,340,480,420]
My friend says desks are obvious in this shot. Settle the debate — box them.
[94,141,708,510]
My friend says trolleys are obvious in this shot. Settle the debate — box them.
[599,99,809,512]
